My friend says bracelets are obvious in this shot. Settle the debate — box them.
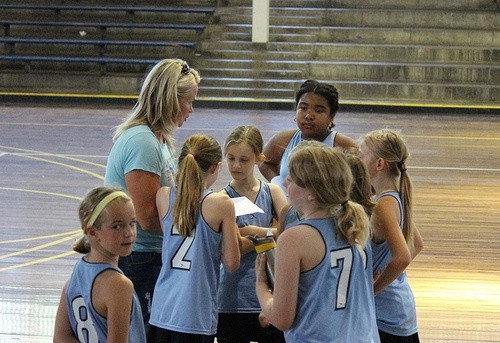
[259,288,271,294]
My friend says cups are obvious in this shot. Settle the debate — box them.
[246,233,277,285]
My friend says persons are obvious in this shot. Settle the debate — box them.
[54,187,146,343]
[354,129,424,343]
[155,134,241,343]
[104,58,201,342]
[259,79,359,198]
[217,125,291,343]
[255,140,382,343]
